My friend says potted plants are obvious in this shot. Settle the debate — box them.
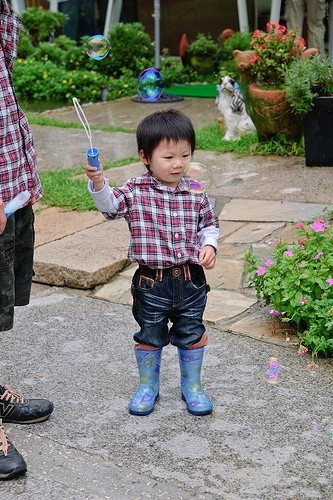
[284,52,333,167]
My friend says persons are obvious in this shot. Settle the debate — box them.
[284,0,326,53]
[85,105,219,417]
[60,0,96,42]
[0,0,53,482]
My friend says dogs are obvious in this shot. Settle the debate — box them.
[214,74,257,142]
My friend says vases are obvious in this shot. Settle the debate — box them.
[246,82,304,143]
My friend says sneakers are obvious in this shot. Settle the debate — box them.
[0,419,27,480]
[0,385,55,424]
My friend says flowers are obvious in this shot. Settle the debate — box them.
[247,20,307,90]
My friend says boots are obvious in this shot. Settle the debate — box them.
[179,347,213,415]
[129,344,163,416]
